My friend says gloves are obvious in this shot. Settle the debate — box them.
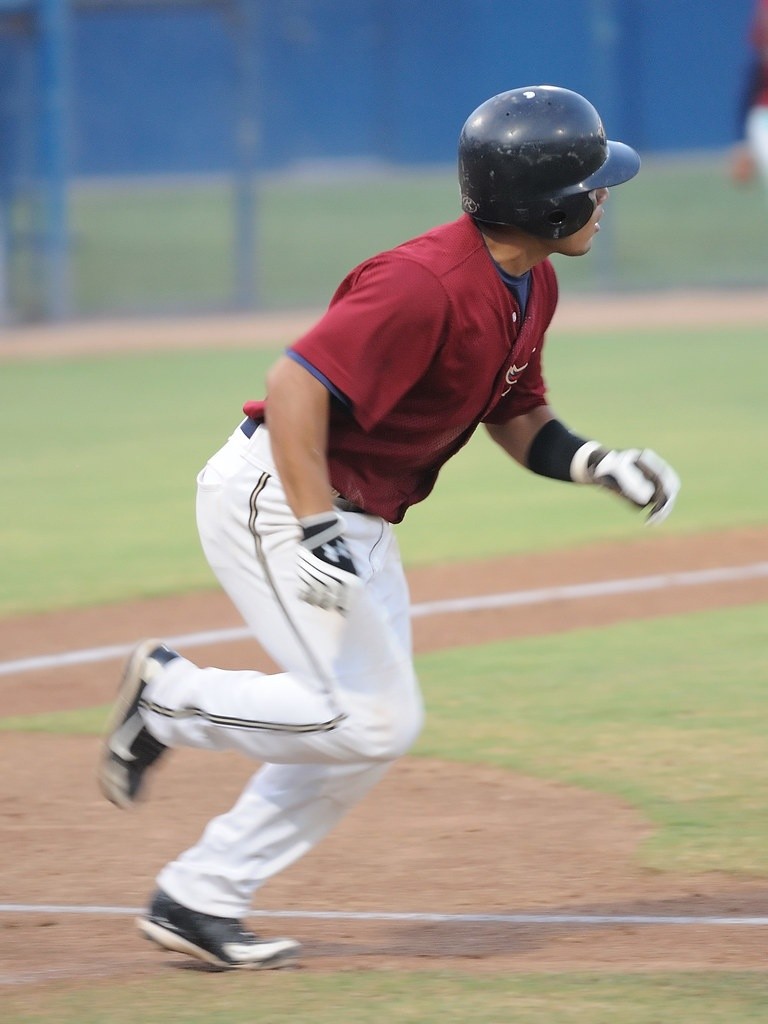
[568,439,680,528]
[292,510,363,620]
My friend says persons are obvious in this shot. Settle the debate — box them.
[94,83,681,971]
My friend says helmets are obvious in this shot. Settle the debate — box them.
[457,85,642,239]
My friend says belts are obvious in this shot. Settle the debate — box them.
[241,417,258,437]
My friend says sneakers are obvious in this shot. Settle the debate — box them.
[94,637,182,810]
[135,888,305,970]
